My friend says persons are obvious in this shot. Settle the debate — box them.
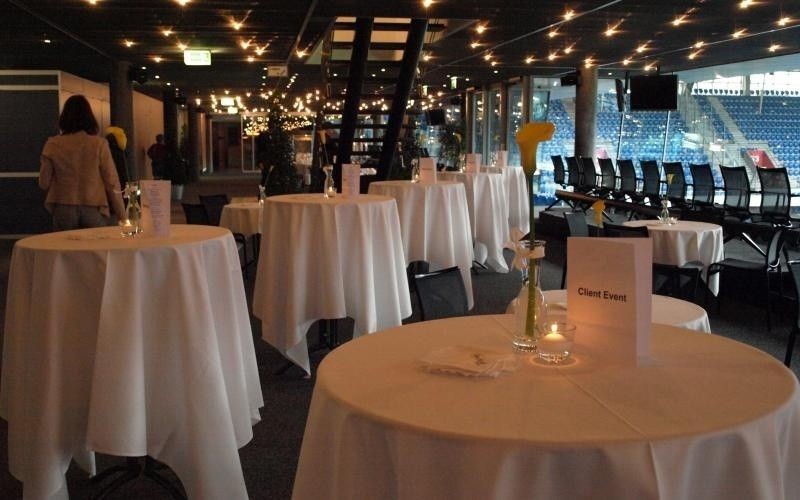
[255,120,273,203]
[147,134,170,180]
[39,95,127,231]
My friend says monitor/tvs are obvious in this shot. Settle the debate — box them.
[615,78,627,112]
[630,75,678,112]
[424,111,429,125]
[429,109,444,126]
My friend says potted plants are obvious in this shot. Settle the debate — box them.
[162,136,188,201]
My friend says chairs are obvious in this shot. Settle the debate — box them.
[706,223,787,335]
[784,306,800,370]
[198,193,245,240]
[544,90,800,289]
[413,265,468,320]
[180,202,247,270]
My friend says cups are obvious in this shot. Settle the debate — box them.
[117,219,138,235]
[328,188,337,198]
[463,161,500,172]
[669,217,679,225]
[538,321,577,361]
[411,175,419,183]
[258,199,264,205]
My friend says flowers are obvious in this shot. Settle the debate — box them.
[454,132,464,168]
[261,164,274,189]
[412,134,425,174]
[515,120,556,338]
[103,126,144,225]
[317,129,338,191]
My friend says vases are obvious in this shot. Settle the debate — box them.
[322,165,337,197]
[459,156,466,172]
[410,158,419,182]
[258,184,267,205]
[120,183,144,238]
[514,241,547,354]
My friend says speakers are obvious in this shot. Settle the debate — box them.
[560,76,566,86]
[566,74,577,85]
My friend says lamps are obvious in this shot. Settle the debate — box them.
[615,69,631,114]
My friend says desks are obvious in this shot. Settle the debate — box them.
[545,290,709,334]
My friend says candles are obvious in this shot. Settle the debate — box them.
[541,323,568,354]
